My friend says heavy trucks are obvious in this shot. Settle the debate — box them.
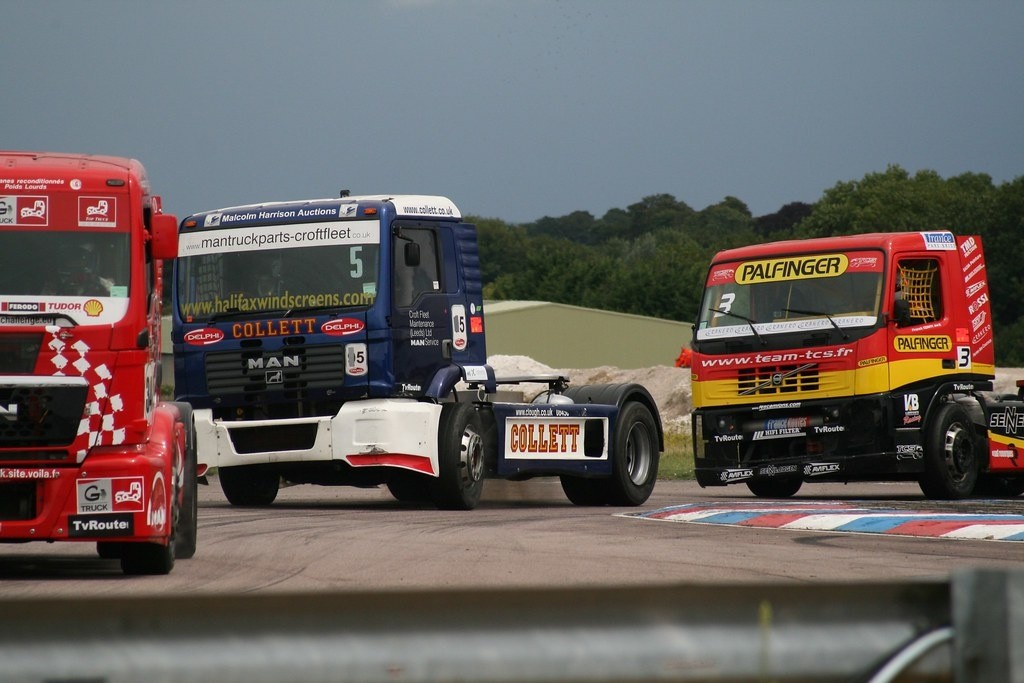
[674,230,1024,499]
[0,153,199,576]
[172,191,664,510]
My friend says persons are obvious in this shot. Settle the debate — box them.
[869,271,909,309]
[25,239,115,298]
